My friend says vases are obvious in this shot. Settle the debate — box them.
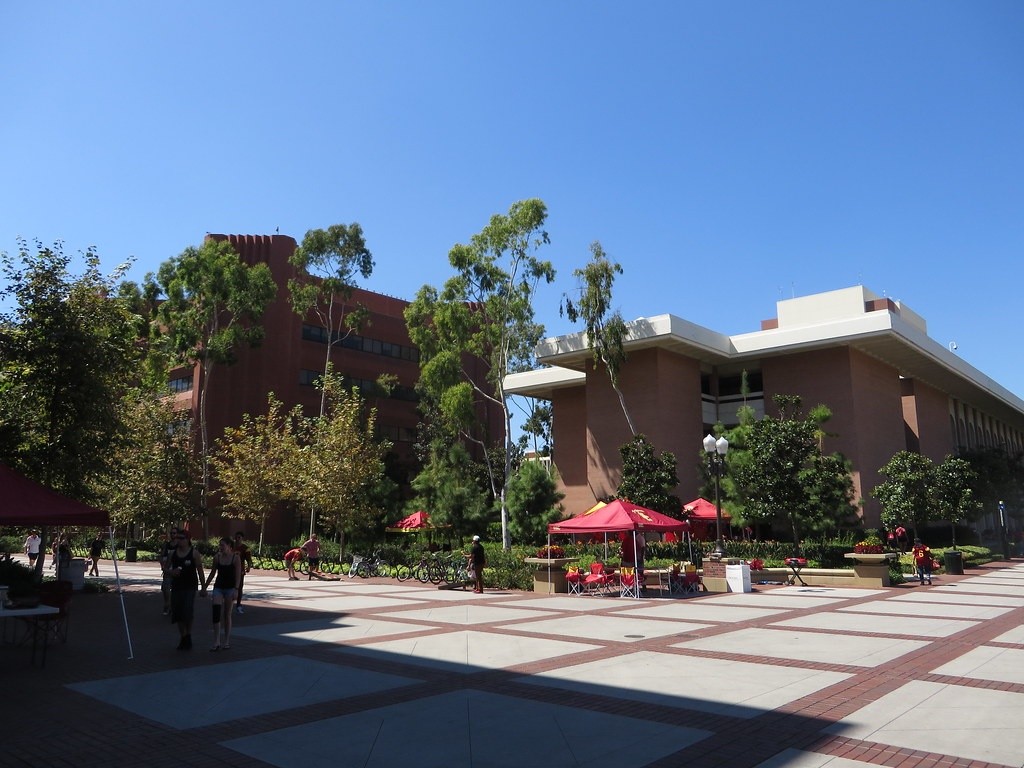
[844,553,897,564]
[524,558,581,569]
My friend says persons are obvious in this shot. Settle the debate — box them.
[463,535,485,593]
[284,534,321,581]
[25,529,41,565]
[159,527,252,652]
[89,531,106,576]
[887,523,908,555]
[622,531,634,567]
[401,541,409,552]
[912,538,934,585]
[636,532,645,574]
[49,537,67,569]
[429,542,438,553]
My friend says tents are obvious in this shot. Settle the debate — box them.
[547,499,693,600]
[681,497,733,544]
[0,464,135,657]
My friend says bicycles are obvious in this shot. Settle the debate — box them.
[300,551,335,575]
[428,554,461,585]
[341,553,359,578]
[397,557,430,583]
[356,546,391,578]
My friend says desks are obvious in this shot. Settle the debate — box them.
[785,564,808,586]
[0,605,59,666]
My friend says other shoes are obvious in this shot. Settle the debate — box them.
[89,573,94,576]
[235,606,244,615]
[920,582,924,585]
[288,577,294,581]
[159,605,170,615]
[473,589,483,593]
[178,636,193,650]
[928,579,932,585]
[96,575,99,577]
[294,577,299,580]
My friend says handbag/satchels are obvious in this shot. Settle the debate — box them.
[931,560,940,572]
[912,566,918,578]
[86,558,92,565]
[468,568,477,579]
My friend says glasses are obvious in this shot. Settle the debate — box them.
[177,536,189,540]
[170,532,178,535]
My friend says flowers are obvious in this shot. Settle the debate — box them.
[537,544,565,559]
[854,539,887,554]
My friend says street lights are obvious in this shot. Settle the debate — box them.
[702,433,728,557]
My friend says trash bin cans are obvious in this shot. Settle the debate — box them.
[945,551,964,573]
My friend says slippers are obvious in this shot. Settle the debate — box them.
[208,644,220,654]
[222,641,231,650]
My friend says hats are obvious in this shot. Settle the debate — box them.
[97,531,102,536]
[178,529,193,544]
[473,535,480,541]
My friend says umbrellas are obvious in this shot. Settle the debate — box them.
[385,511,452,559]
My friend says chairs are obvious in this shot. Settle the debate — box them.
[672,563,702,596]
[14,581,73,648]
[565,564,643,599]
